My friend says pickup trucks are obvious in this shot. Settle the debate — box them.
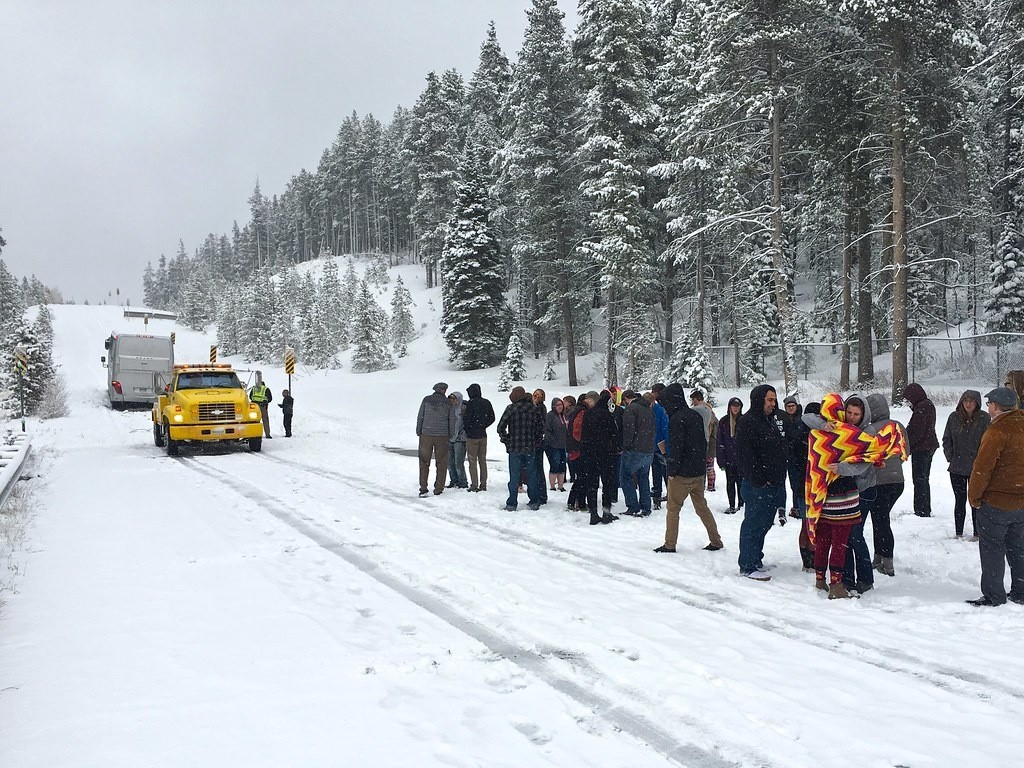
[151,363,263,457]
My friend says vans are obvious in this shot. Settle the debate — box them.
[101,331,174,410]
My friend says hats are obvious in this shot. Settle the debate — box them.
[729,399,742,406]
[985,387,1018,406]
[620,389,636,406]
[784,397,797,406]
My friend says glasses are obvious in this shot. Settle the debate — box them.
[1005,382,1011,387]
[986,402,991,406]
[787,404,796,408]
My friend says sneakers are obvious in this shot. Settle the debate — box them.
[703,544,719,551]
[748,572,771,581]
[653,545,676,553]
[761,564,775,570]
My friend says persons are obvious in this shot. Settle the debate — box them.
[653,383,724,553]
[736,384,787,580]
[545,397,569,492]
[497,386,548,511]
[781,395,804,519]
[463,384,495,493]
[901,382,940,517]
[553,396,576,484]
[565,391,600,512]
[580,394,623,525]
[444,391,469,489]
[277,389,294,437]
[964,370,1024,607]
[609,386,656,518]
[642,383,670,510]
[942,390,992,542]
[716,397,745,514]
[249,381,272,439]
[800,393,907,601]
[416,382,455,497]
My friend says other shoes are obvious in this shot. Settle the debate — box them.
[1005,592,1024,604]
[528,503,539,510]
[789,507,801,520]
[954,535,963,539]
[725,506,734,514]
[517,486,526,493]
[285,434,292,437]
[965,598,994,607]
[707,488,715,492]
[971,536,979,542]
[419,483,487,498]
[267,436,272,439]
[502,505,517,512]
[732,508,740,513]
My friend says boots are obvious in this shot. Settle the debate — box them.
[550,479,667,525]
[778,507,787,525]
[802,554,895,600]
